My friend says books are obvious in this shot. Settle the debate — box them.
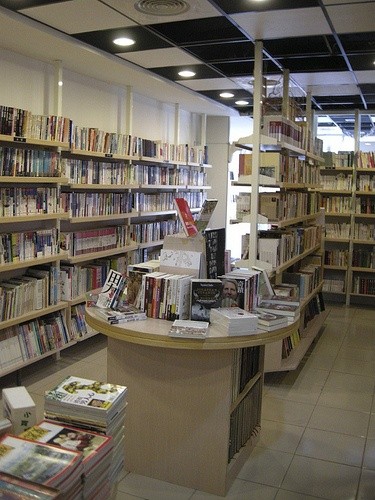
[0,105,375,374]
[0,376,132,500]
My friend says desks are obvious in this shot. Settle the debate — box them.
[84,288,301,497]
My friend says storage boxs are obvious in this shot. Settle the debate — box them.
[2,386,37,435]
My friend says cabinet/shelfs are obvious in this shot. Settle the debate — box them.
[0,132,375,380]
